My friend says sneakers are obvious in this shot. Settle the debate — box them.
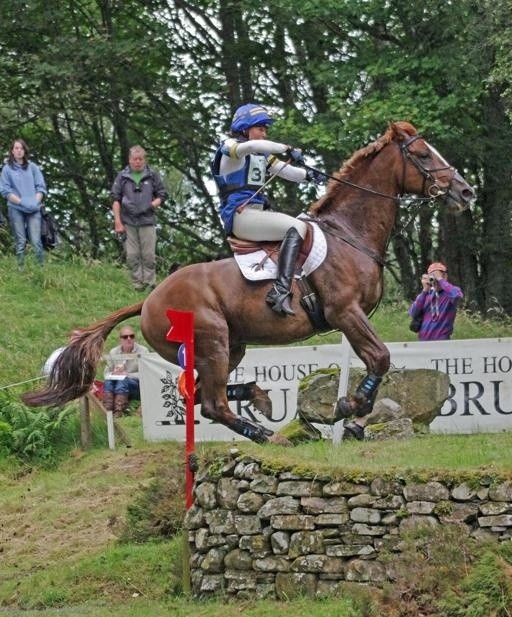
[132,281,153,292]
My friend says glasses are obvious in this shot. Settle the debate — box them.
[120,334,135,340]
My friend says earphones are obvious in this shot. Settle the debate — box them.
[241,129,249,138]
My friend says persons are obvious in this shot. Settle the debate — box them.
[1,137,49,270]
[111,143,169,294]
[42,327,104,399]
[210,103,330,317]
[102,324,151,417]
[407,260,464,340]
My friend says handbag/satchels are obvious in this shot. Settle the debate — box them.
[409,311,424,332]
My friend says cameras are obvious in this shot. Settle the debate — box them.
[426,274,436,285]
[115,232,127,243]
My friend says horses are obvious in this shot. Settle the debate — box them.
[17,113,479,446]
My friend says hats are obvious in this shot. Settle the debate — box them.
[427,261,448,274]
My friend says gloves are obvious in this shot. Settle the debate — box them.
[286,148,306,166]
[305,169,324,183]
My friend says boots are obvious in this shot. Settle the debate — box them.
[112,392,129,418]
[264,226,306,317]
[103,391,114,411]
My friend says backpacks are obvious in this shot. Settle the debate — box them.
[23,200,57,250]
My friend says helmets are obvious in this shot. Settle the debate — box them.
[230,102,276,133]
